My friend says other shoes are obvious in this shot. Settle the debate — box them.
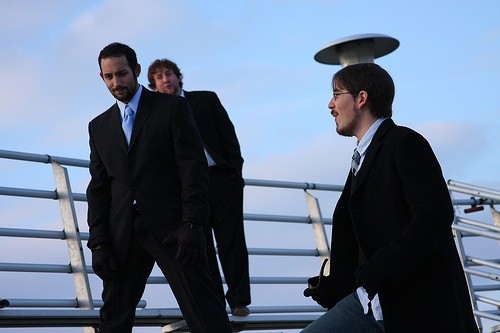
[231,306,250,316]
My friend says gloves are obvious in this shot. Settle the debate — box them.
[164,222,207,268]
[92,246,116,282]
[303,275,350,308]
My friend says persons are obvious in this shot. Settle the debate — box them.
[300,62,480,333]
[147,58,251,317]
[84,41,233,333]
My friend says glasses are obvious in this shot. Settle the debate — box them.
[333,91,350,100]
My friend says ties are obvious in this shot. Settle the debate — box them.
[122,105,134,147]
[351,148,361,175]
